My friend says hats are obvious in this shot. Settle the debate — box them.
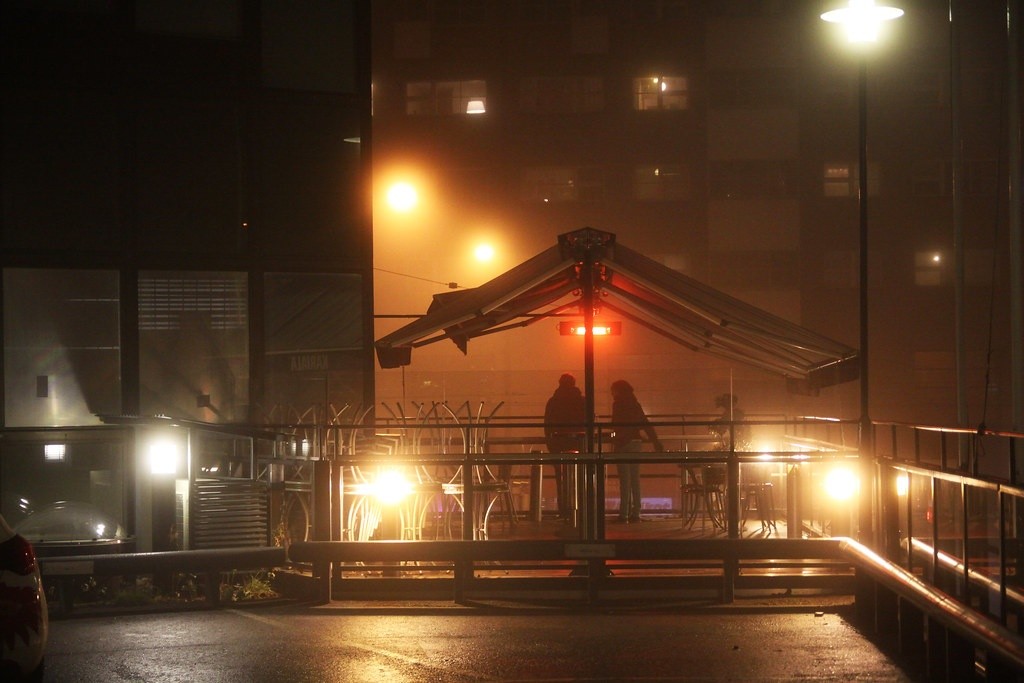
[560,373,575,386]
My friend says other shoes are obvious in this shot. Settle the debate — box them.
[558,509,574,519]
[614,518,629,524]
[631,517,641,523]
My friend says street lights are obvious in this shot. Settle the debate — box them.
[818,0,907,610]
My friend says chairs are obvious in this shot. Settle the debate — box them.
[254,401,519,575]
[680,459,776,533]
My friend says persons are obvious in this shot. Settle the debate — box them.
[610,380,662,522]
[545,373,591,520]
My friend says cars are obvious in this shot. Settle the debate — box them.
[0,511,50,683]
